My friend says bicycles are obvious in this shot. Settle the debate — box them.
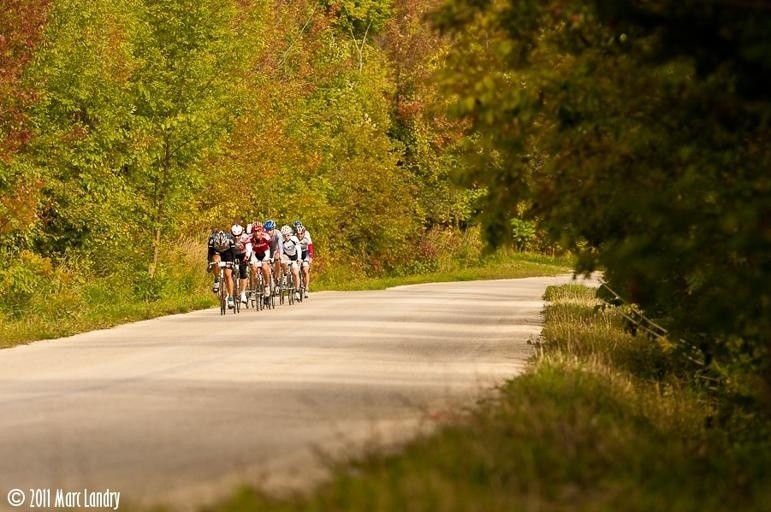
[207,258,307,315]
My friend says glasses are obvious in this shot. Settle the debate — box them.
[297,233,303,235]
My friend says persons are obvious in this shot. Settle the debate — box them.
[206,218,314,308]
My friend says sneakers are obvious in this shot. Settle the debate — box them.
[213,278,220,293]
[264,285,272,298]
[240,291,248,303]
[275,282,281,295]
[250,291,256,301]
[294,290,310,301]
[228,297,235,309]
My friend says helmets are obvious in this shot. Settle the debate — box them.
[213,230,228,246]
[281,225,293,235]
[232,225,242,235]
[294,221,306,233]
[245,220,277,235]
